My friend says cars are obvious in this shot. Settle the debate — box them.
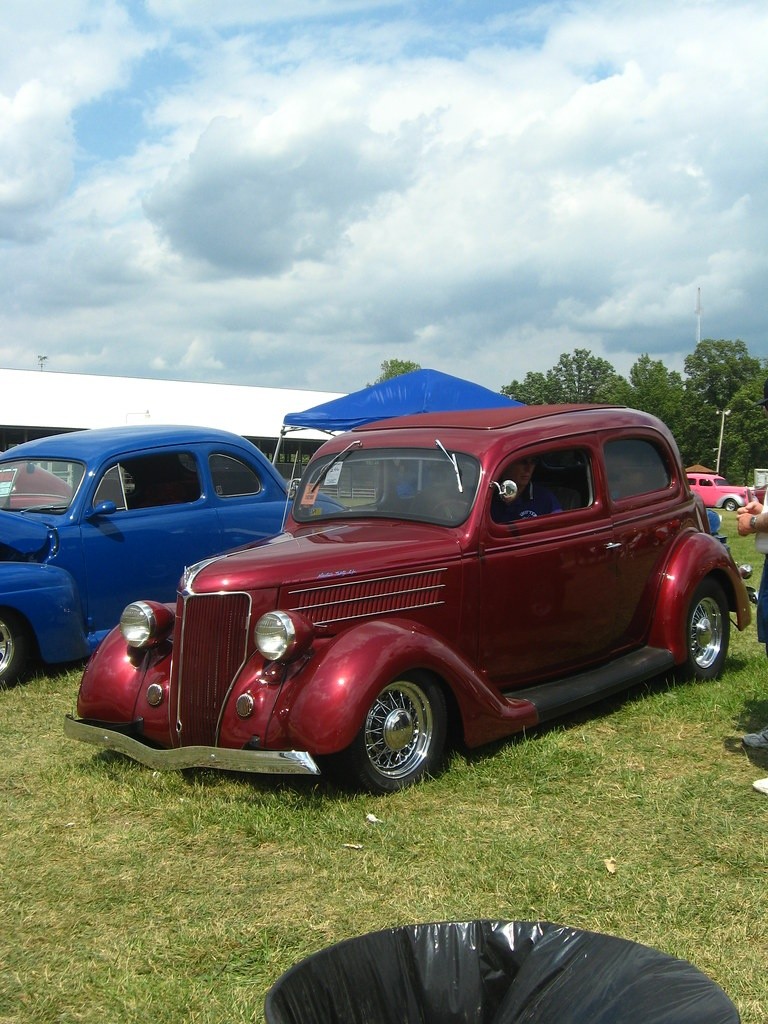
[686,472,753,512]
[0,421,349,698]
[62,400,754,803]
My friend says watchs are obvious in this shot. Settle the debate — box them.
[750,515,758,532]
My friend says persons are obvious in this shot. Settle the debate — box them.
[734,480,767,797]
[489,453,564,526]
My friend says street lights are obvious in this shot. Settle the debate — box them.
[715,407,732,476]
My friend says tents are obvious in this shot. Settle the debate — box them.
[267,368,527,466]
